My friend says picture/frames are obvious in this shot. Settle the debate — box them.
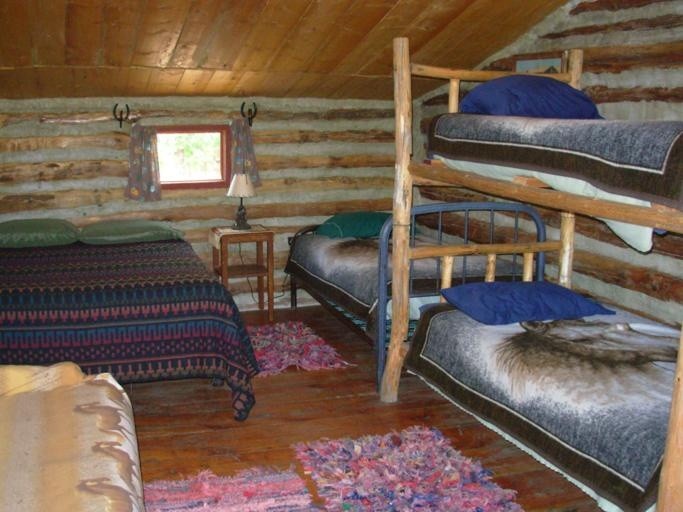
[512,50,567,74]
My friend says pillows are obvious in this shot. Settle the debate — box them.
[315,212,423,240]
[440,280,615,325]
[0,219,186,248]
[460,75,605,119]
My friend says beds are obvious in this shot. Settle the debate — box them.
[0,362,145,512]
[285,203,546,393]
[0,240,260,422]
[380,36,683,512]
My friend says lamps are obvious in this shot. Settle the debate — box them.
[226,173,257,230]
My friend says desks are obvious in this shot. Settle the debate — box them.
[208,224,275,322]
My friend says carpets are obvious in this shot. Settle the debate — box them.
[290,425,526,512]
[143,464,323,512]
[247,321,358,379]
